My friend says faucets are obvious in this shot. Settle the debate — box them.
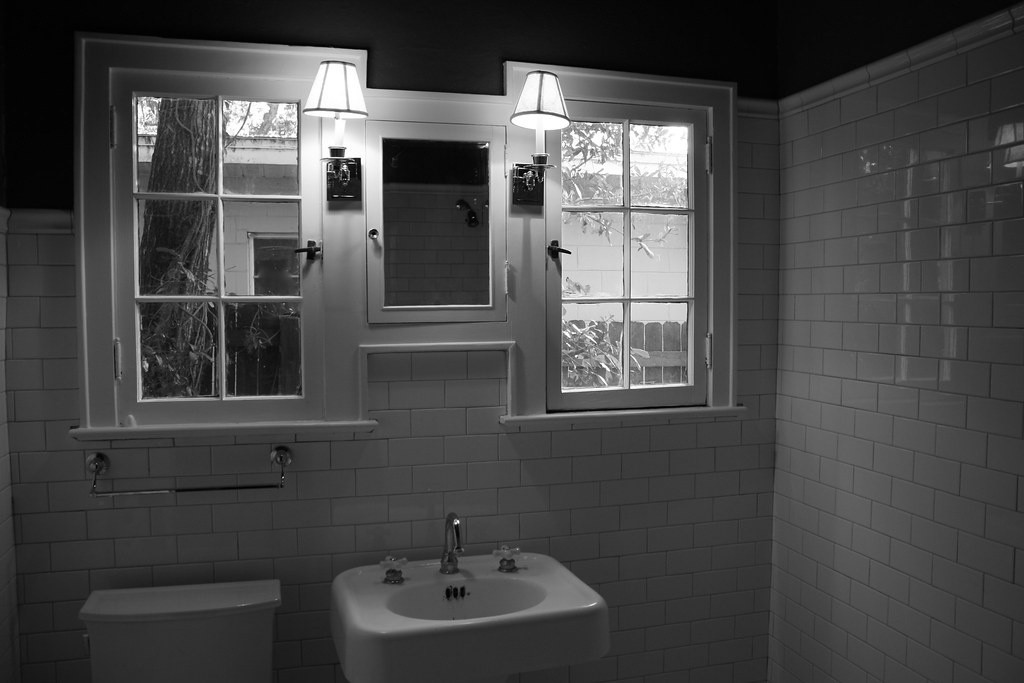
[438,512,465,574]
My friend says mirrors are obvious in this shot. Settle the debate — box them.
[364,120,508,325]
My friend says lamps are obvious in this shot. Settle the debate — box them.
[303,61,368,206]
[510,70,571,206]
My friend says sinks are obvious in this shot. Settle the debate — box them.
[328,551,611,683]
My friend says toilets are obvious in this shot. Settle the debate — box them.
[78,577,283,683]
